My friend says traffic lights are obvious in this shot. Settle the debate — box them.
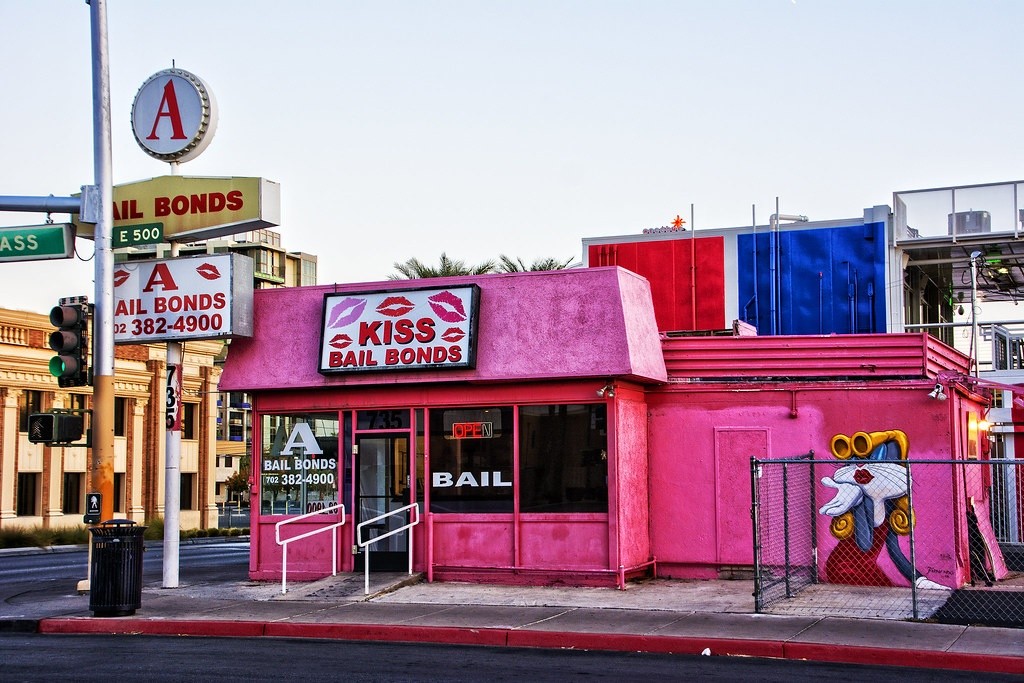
[28,414,59,442]
[48,305,81,380]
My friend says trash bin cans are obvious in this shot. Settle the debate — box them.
[88,519,148,617]
